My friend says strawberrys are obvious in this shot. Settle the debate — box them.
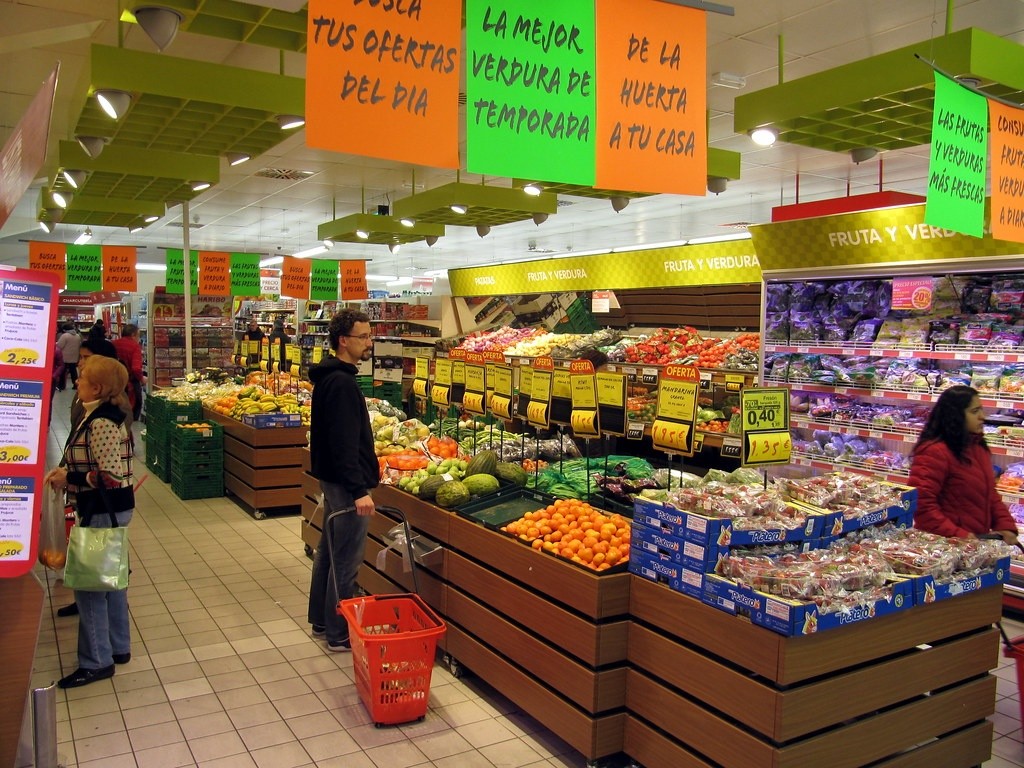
[678,477,976,606]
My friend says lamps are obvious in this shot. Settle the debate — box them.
[40,8,305,234]
[74,226,93,245]
[322,182,629,255]
[747,126,779,146]
[706,177,727,194]
[850,148,879,164]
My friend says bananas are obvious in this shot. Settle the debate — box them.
[230,387,311,425]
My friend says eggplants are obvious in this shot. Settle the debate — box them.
[593,461,664,496]
[479,436,580,466]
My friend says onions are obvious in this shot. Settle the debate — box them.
[462,324,532,352]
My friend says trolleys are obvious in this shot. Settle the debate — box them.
[980,534,1024,737]
[325,505,448,729]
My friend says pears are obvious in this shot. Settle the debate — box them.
[400,457,468,494]
[370,414,430,455]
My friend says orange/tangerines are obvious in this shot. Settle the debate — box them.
[500,498,631,571]
[176,423,211,431]
[215,396,238,416]
[377,437,458,478]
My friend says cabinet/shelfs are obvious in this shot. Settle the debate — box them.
[299,317,441,403]
[756,267,1024,615]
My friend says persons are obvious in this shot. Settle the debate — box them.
[43,355,137,688]
[564,348,618,458]
[243,320,290,371]
[48,319,148,615]
[307,308,380,652]
[906,385,1019,547]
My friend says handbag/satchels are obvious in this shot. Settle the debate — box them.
[62,511,129,592]
[38,482,68,578]
[75,483,135,518]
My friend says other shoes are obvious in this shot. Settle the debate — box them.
[58,602,79,617]
[327,636,352,651]
[311,623,326,635]
[71,384,77,389]
[60,387,65,390]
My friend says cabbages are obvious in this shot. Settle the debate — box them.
[696,405,725,422]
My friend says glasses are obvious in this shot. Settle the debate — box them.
[348,333,373,340]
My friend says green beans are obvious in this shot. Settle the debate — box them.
[459,431,529,454]
[527,458,651,498]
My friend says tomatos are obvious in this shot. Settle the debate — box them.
[627,395,713,433]
[696,419,728,434]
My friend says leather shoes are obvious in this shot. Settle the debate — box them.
[112,653,131,664]
[58,664,115,688]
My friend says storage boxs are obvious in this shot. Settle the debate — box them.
[241,411,301,429]
[143,392,224,501]
[355,375,403,410]
[627,476,1011,638]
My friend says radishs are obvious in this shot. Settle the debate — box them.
[459,420,481,430]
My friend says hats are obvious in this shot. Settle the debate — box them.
[274,320,284,328]
[88,319,107,344]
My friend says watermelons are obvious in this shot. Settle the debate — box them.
[419,449,527,506]
